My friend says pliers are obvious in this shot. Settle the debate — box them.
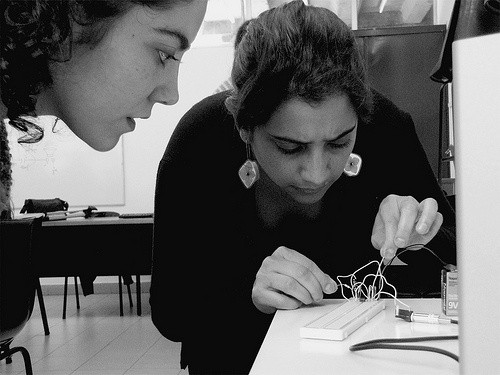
[47,206,97,220]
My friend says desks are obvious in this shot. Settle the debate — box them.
[42,218,154,319]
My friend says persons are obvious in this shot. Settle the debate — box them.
[149,0,456,375]
[0,0,208,342]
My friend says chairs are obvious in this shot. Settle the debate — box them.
[0,222,37,375]
